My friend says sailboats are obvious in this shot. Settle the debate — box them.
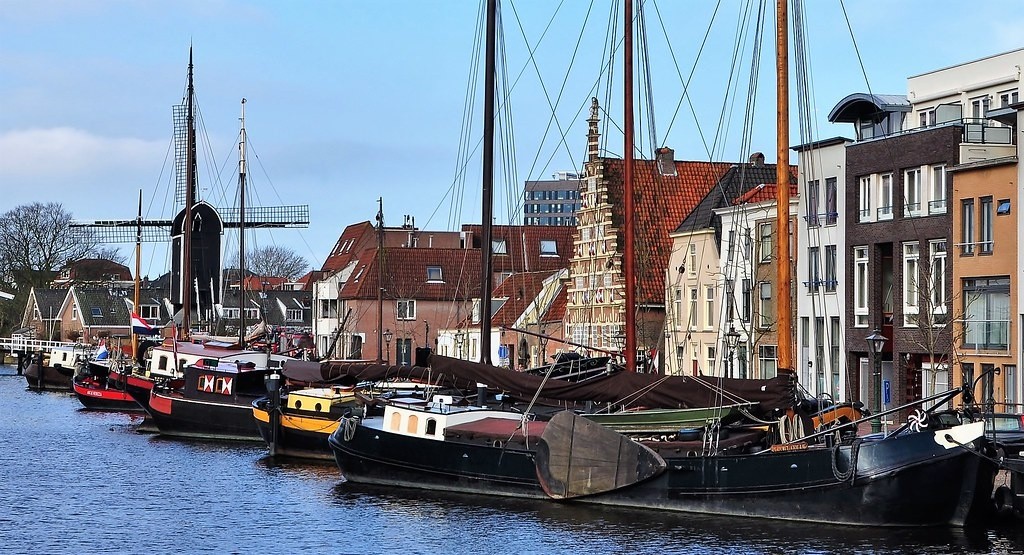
[20,0,1024,541]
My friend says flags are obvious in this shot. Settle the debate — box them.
[97,339,108,359]
[132,312,161,335]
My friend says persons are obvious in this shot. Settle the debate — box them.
[475,380,488,408]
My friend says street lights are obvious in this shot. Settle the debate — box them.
[722,323,742,378]
[538,329,549,366]
[383,328,394,365]
[614,330,626,363]
[455,329,465,360]
[864,324,890,434]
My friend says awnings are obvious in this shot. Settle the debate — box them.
[998,203,1010,212]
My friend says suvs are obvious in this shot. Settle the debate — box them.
[931,409,1024,461]
